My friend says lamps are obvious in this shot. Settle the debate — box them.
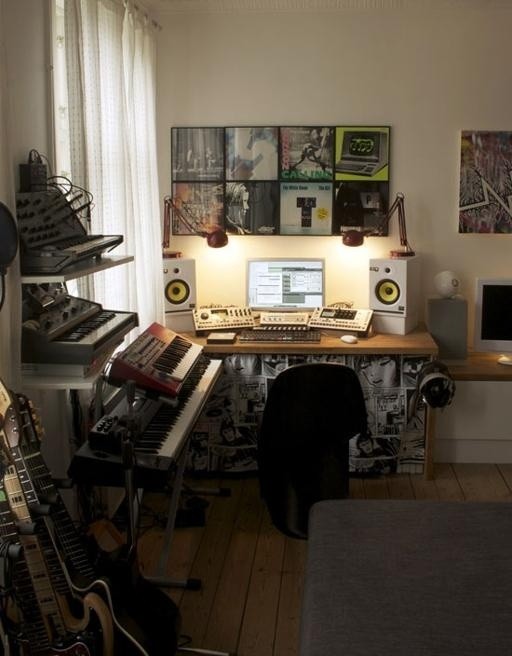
[342,192,415,257]
[161,197,228,257]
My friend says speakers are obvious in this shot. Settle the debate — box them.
[425,296,470,363]
[157,254,197,335]
[369,256,422,337]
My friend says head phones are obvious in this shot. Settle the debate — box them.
[418,369,456,413]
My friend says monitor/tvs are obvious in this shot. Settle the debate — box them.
[472,277,512,354]
[245,258,325,313]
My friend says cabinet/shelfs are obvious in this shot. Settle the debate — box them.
[21,256,134,390]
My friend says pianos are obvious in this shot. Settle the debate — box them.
[67,358,223,481]
[21,234,124,274]
[24,295,139,361]
[108,320,203,394]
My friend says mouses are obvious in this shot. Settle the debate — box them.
[340,334,357,344]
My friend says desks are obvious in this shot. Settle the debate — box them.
[303,499,512,655]
[426,351,512,483]
[176,321,438,482]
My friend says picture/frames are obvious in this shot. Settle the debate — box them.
[171,127,225,182]
[277,181,335,236]
[223,126,280,182]
[334,125,390,182]
[278,126,335,182]
[171,182,226,236]
[331,180,389,237]
[223,180,278,235]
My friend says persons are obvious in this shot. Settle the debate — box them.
[292,143,327,171]
[225,182,250,235]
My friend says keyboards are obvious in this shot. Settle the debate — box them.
[239,328,322,345]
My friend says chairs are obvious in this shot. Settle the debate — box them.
[247,362,368,538]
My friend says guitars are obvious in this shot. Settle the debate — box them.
[29,399,182,656]
[1,379,115,656]
[12,392,150,656]
